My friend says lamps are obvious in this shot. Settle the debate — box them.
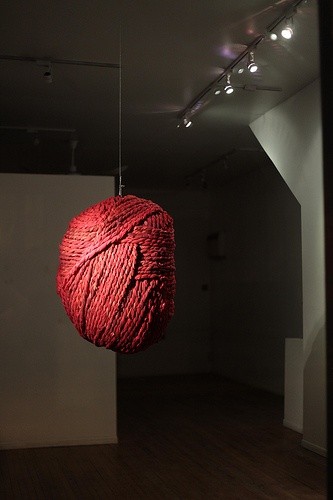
[184,116,192,128]
[224,74,234,95]
[247,53,258,73]
[281,16,296,40]
[214,88,221,95]
